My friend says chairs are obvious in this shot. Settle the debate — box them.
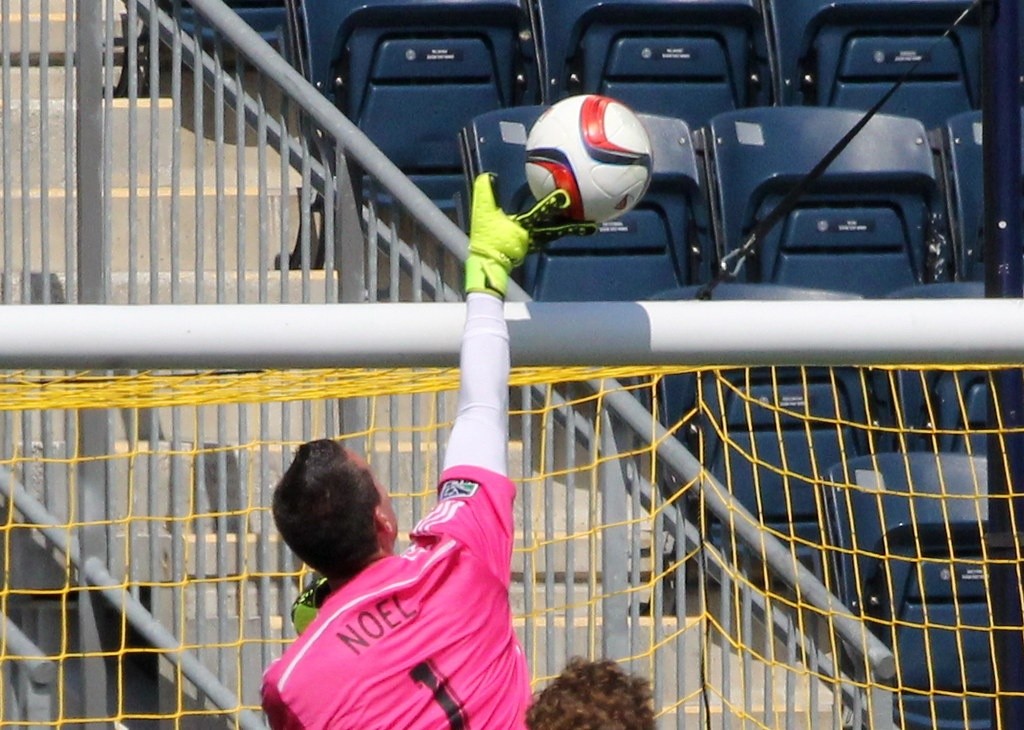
[98,0,1024,730]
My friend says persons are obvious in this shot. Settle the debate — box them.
[259,171,599,730]
[524,654,659,730]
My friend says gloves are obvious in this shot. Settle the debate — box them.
[290,576,329,636]
[464,172,598,299]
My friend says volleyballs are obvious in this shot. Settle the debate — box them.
[526,93,654,225]
